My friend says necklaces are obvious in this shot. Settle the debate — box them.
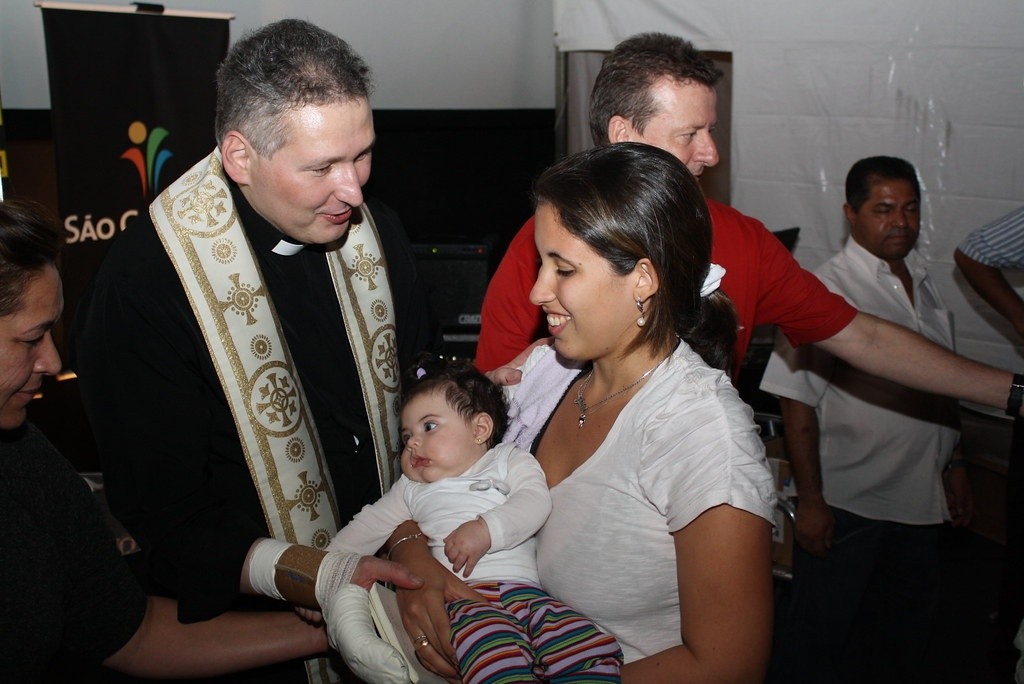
[573,338,682,428]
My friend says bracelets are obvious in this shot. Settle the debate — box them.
[1005,373,1024,417]
[386,533,421,560]
[947,459,968,468]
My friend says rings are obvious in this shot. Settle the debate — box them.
[414,636,429,649]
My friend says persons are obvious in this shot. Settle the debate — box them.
[78,19,554,684]
[297,360,624,684]
[0,199,331,684]
[954,206,1024,674]
[392,143,778,684]
[758,156,973,684]
[475,34,1024,419]
